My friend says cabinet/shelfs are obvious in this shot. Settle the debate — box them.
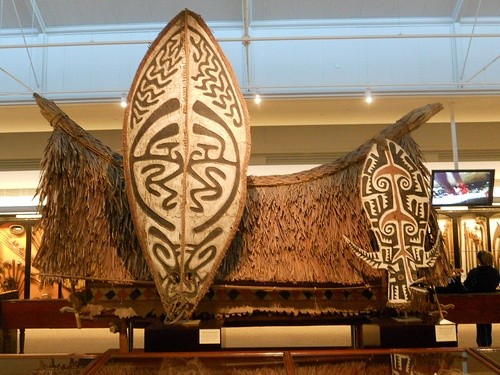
[143,318,222,350]
[379,316,457,348]
[79,348,500,375]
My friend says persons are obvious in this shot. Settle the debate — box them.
[464,250,500,346]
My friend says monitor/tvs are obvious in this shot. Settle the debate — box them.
[430,169,495,205]
